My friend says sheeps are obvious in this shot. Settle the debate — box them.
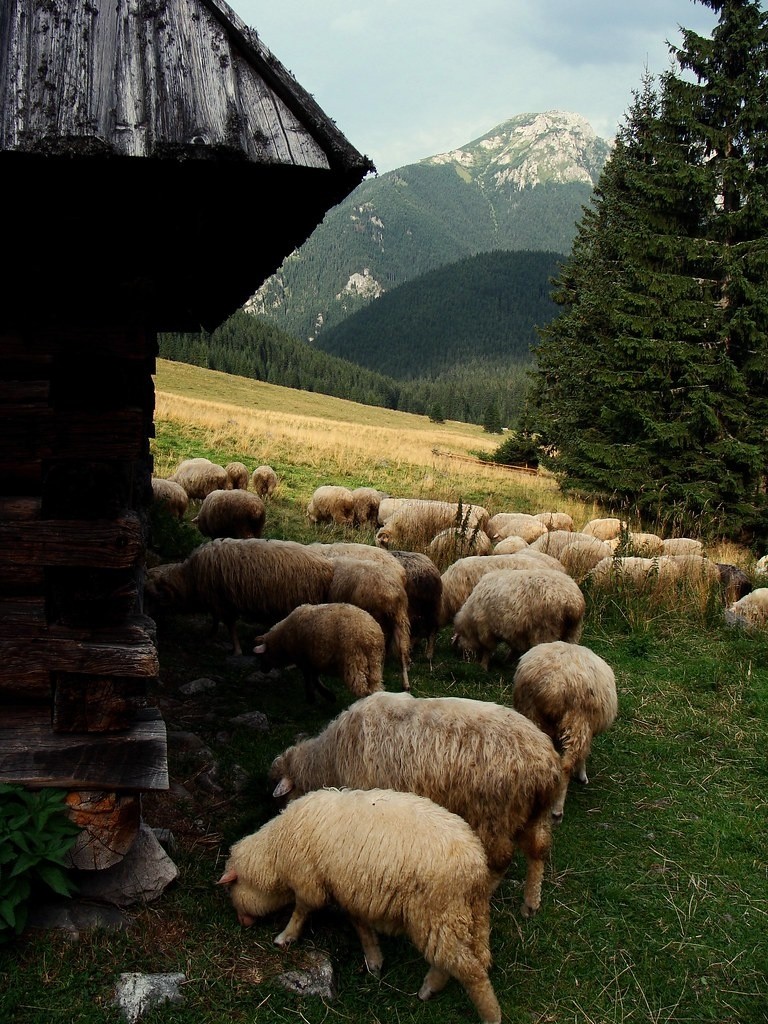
[253,603,386,703]
[514,640,620,823]
[145,457,278,539]
[214,784,504,1024]
[267,692,563,916]
[141,485,768,691]
